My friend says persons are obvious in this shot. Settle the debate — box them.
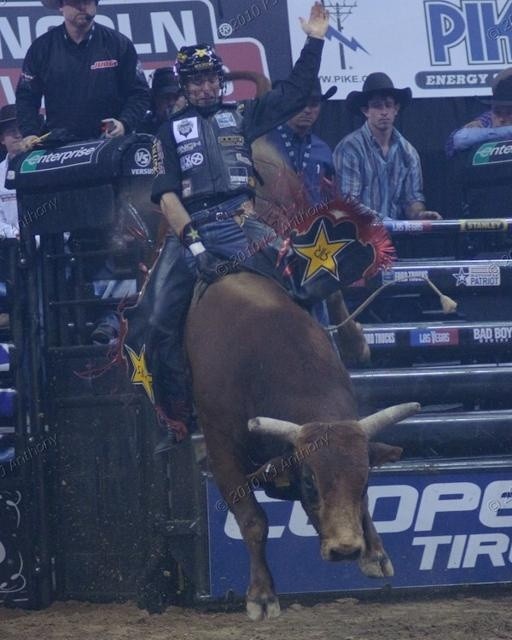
[428,68,512,251]
[0,106,45,287]
[143,2,340,461]
[16,1,150,154]
[138,64,190,139]
[260,71,335,213]
[330,69,444,259]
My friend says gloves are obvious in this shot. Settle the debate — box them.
[193,250,229,285]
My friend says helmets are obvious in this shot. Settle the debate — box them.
[172,41,227,115]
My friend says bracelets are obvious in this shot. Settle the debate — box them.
[178,221,204,246]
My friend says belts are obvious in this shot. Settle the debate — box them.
[193,207,247,235]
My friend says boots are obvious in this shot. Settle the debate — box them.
[149,395,197,454]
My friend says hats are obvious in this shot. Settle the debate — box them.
[0,105,44,129]
[345,71,414,116]
[307,73,340,103]
[478,66,512,107]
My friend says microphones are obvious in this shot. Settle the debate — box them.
[86,14,95,20]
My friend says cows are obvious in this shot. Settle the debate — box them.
[147,69,423,623]
